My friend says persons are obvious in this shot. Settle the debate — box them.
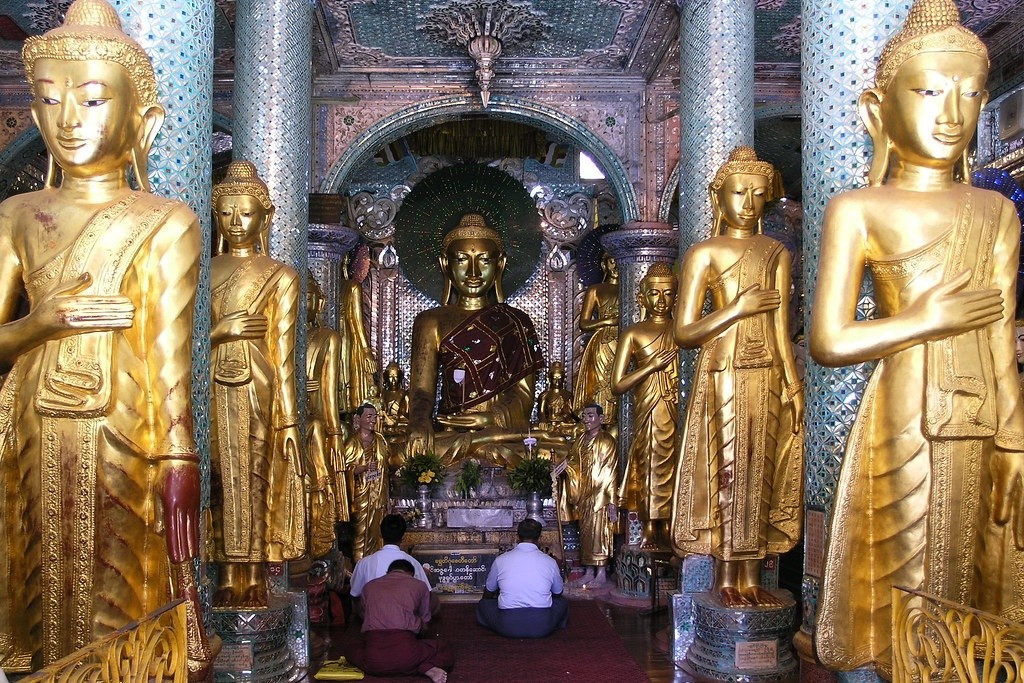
[349,513,440,623]
[807,0,1024,683]
[207,161,302,608]
[383,210,567,459]
[344,560,454,683]
[308,242,388,564]
[673,149,807,607]
[569,251,682,588]
[0,0,211,683]
[476,519,570,638]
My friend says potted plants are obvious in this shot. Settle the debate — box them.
[455,460,483,499]
[507,464,527,499]
[518,456,555,527]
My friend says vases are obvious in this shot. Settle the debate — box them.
[418,485,432,499]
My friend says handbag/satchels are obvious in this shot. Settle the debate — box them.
[314,656,364,681]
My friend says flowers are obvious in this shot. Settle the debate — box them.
[392,442,450,489]
